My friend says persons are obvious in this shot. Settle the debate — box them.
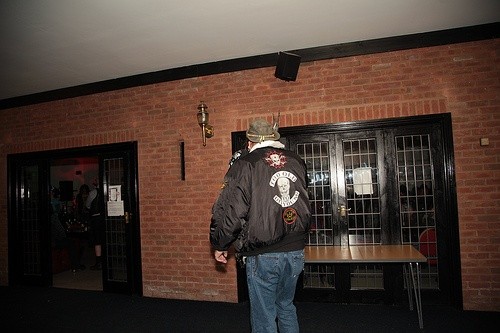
[84,178,103,271]
[209,118,312,333]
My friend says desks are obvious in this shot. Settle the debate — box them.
[264,244,428,330]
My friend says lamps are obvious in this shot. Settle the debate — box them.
[274,52,301,83]
[197,100,213,149]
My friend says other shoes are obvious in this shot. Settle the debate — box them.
[89,265,101,271]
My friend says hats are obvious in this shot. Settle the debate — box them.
[246,120,280,143]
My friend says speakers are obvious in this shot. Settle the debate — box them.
[275,51,301,82]
